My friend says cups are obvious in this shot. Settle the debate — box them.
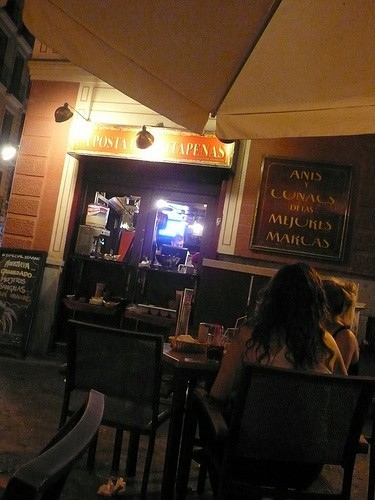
[207,334,227,360]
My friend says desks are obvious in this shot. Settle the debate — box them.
[62,298,177,335]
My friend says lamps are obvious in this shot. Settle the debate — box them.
[135,122,164,149]
[54,103,89,123]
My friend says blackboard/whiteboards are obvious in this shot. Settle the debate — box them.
[0,248,48,351]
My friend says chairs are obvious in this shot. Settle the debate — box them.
[0,389,105,500]
[58,319,173,498]
[176,362,375,500]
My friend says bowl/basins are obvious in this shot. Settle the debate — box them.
[168,336,207,354]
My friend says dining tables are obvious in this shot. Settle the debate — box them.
[140,341,225,500]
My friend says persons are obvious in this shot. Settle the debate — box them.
[172,235,184,249]
[321,279,359,376]
[209,262,346,493]
